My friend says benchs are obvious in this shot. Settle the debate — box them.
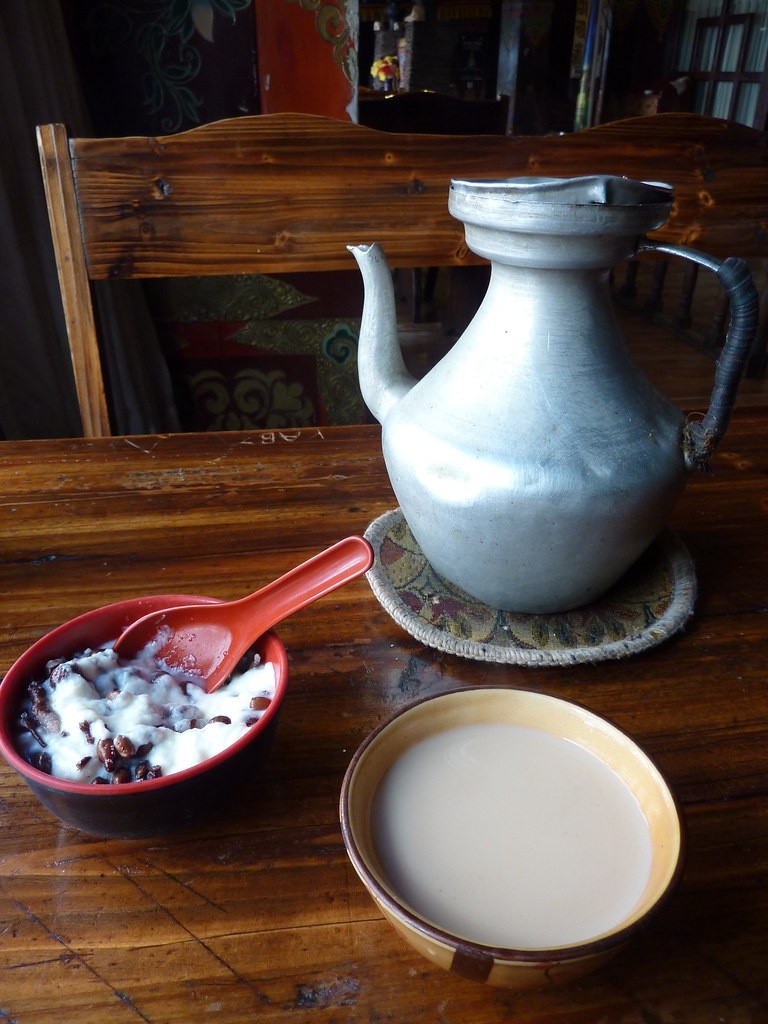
[34,112,768,436]
[359,93,512,324]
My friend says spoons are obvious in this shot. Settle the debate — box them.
[112,534,376,694]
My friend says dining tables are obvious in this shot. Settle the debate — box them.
[0,402,768,1024]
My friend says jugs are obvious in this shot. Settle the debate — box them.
[346,173,757,616]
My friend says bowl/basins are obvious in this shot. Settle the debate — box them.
[339,684,685,994]
[0,593,290,835]
